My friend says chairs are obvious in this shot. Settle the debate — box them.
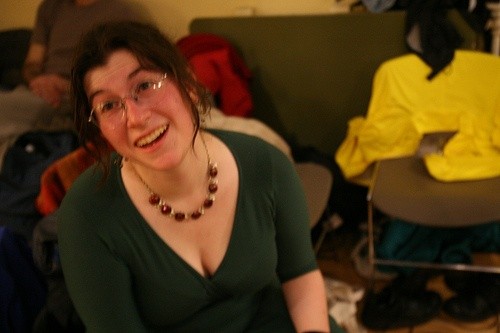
[367,133,500,292]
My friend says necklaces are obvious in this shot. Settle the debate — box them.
[132,129,218,221]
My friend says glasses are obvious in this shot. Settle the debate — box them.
[86,71,167,129]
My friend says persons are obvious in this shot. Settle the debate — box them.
[0,0,154,333]
[58,21,347,333]
